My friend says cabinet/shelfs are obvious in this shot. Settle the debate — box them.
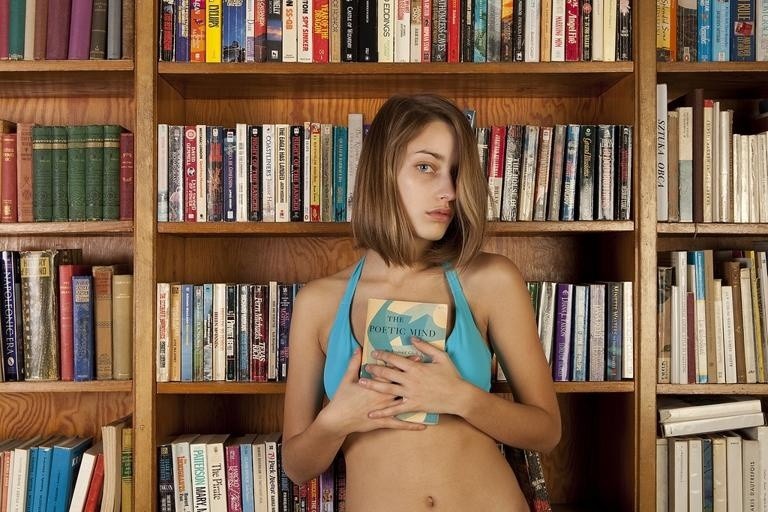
[0,0,761,512]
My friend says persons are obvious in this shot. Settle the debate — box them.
[282,96,560,511]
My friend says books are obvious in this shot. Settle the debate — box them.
[476,125,634,222]
[656,398,766,512]
[158,0,633,63]
[2,1,135,61]
[1,248,132,382]
[505,446,552,511]
[656,2,768,62]
[158,435,346,512]
[496,281,633,384]
[0,118,132,224]
[156,281,305,381]
[158,112,363,222]
[656,249,767,382]
[0,413,133,511]
[656,82,766,222]
[361,300,448,426]
